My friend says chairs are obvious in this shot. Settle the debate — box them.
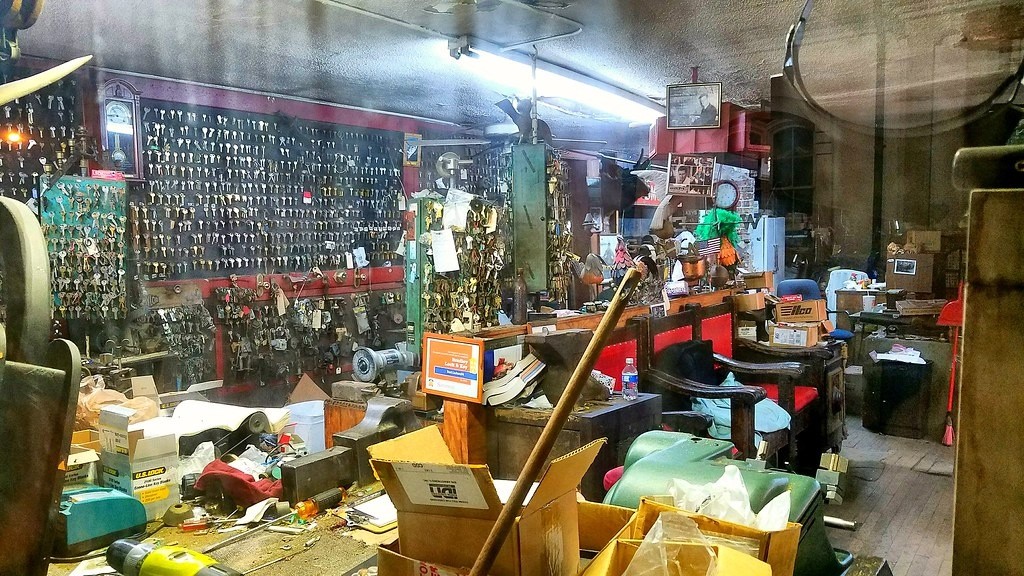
[691,295,834,472]
[580,317,760,507]
[777,279,857,365]
[642,302,802,483]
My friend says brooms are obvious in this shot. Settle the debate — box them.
[941,274,966,449]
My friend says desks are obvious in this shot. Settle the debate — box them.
[38,489,381,576]
[836,280,887,334]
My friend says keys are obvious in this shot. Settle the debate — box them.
[546,156,574,307]
[423,191,505,336]
[1,83,408,378]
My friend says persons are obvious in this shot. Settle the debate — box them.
[670,155,712,195]
[697,93,717,126]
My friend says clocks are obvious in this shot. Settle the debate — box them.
[88,72,145,185]
[712,180,740,210]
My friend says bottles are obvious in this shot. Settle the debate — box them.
[622,358,638,402]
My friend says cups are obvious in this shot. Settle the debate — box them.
[863,296,876,312]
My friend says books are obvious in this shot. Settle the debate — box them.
[480,349,545,406]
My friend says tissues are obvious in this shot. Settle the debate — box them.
[662,259,690,297]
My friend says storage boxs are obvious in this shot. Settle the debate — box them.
[376,500,638,576]
[95,402,184,524]
[904,228,943,254]
[363,421,636,574]
[64,447,100,490]
[884,250,944,295]
[70,428,104,455]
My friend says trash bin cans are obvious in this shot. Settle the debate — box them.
[861,358,935,439]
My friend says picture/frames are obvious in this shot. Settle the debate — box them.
[665,83,722,130]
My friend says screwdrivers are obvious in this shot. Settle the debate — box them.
[200,487,347,554]
